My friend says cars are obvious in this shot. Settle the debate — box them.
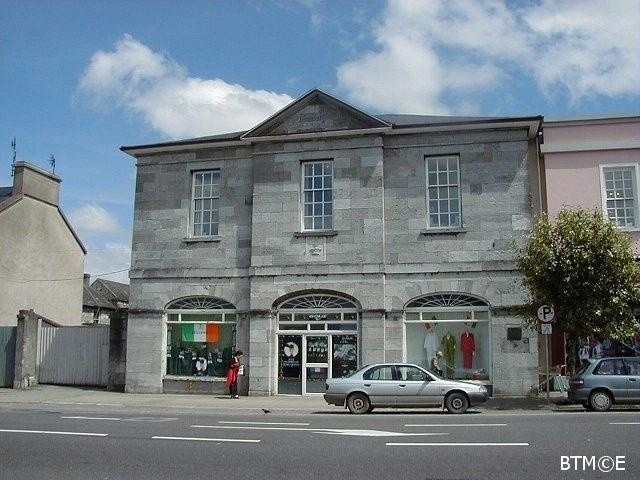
[323,362,489,413]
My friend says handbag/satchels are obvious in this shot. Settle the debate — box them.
[435,369,442,376]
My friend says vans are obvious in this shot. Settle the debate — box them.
[568,356,640,412]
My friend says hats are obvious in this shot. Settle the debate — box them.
[437,351,443,357]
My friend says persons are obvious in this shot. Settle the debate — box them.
[431,350,444,376]
[460,330,475,369]
[559,336,640,376]
[424,330,440,368]
[441,332,457,379]
[226,350,244,398]
